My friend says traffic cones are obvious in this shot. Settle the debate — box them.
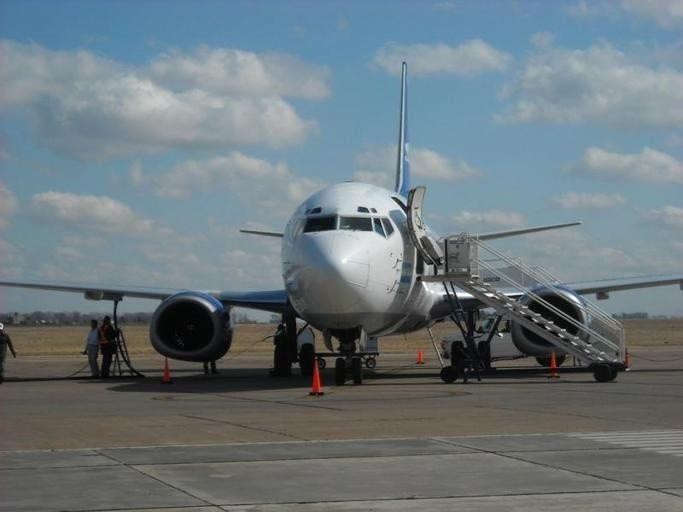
[416,345,425,364]
[309,356,325,396]
[163,355,174,384]
[548,352,561,379]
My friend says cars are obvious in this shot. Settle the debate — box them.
[442,312,590,368]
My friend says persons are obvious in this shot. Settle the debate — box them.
[97,315,116,378]
[272,323,296,378]
[0,322,17,384]
[82,320,100,377]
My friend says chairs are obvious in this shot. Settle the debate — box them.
[501,322,510,333]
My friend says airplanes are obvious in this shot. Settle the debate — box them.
[1,62,683,383]
